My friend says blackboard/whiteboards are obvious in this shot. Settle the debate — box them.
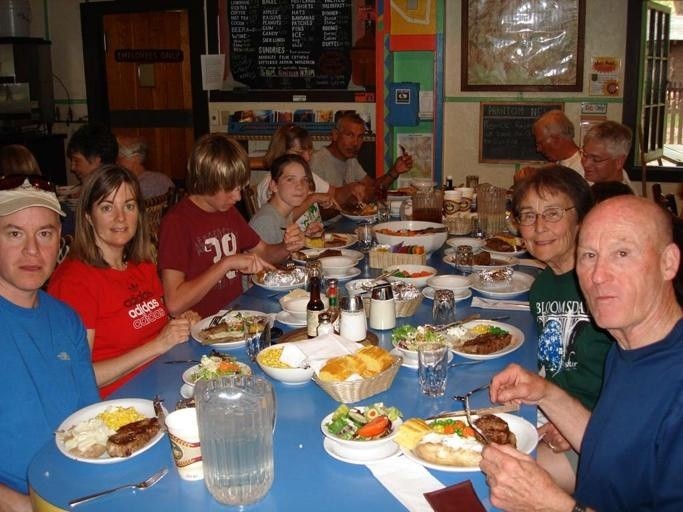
[228,0,353,90]
[480,101,566,165]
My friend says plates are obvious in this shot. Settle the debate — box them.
[55,186,80,195]
[66,199,79,204]
[179,175,539,473]
[55,397,170,465]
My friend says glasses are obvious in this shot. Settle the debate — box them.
[0,171,57,193]
[513,204,577,226]
[334,127,365,138]
[579,149,613,164]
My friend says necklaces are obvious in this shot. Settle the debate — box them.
[110,263,127,267]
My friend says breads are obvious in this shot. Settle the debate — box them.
[356,345,398,376]
[409,441,484,467]
[199,321,245,341]
[59,422,107,459]
[320,356,360,382]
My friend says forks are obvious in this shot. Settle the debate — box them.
[68,467,168,508]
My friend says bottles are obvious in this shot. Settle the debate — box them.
[307,276,395,342]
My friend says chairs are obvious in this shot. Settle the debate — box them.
[652,182,679,218]
[240,184,260,218]
[138,186,179,261]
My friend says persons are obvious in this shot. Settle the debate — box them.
[479,194,682,512]
[47,163,202,400]
[242,109,413,294]
[156,131,304,320]
[510,163,615,497]
[0,174,102,511]
[0,124,175,238]
[532,109,584,179]
[580,120,635,194]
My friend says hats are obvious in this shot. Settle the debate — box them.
[0,177,67,219]
